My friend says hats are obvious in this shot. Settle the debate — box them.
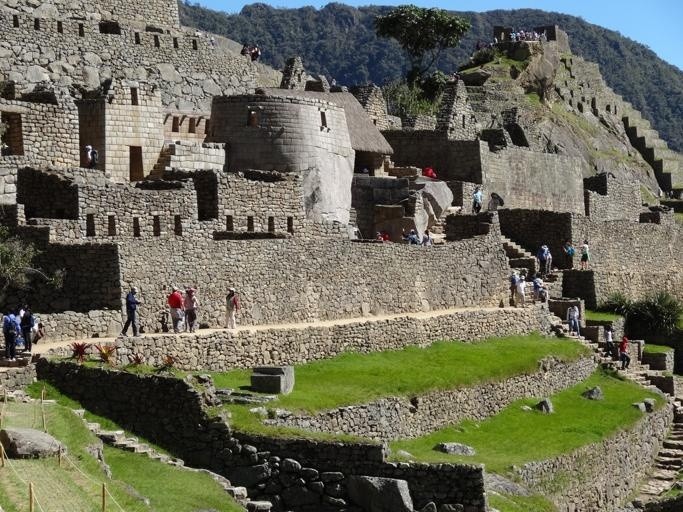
[623,336,628,344]
[172,286,179,292]
[85,144,92,150]
[511,270,526,280]
[131,286,139,293]
[226,286,236,293]
[185,288,197,293]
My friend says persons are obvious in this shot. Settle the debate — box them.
[85,145,94,168]
[0,303,43,363]
[374,231,383,241]
[250,44,261,61]
[192,29,219,47]
[422,163,433,177]
[492,29,547,46]
[183,288,200,333]
[401,229,420,245]
[508,239,631,371]
[168,286,185,333]
[467,187,484,214]
[418,230,432,246]
[221,287,238,329]
[401,228,411,238]
[487,194,499,211]
[119,286,144,336]
[240,43,250,57]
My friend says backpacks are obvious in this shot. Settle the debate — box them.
[91,150,99,165]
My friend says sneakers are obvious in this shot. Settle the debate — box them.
[170,324,238,334]
[119,330,143,339]
[1,347,31,363]
